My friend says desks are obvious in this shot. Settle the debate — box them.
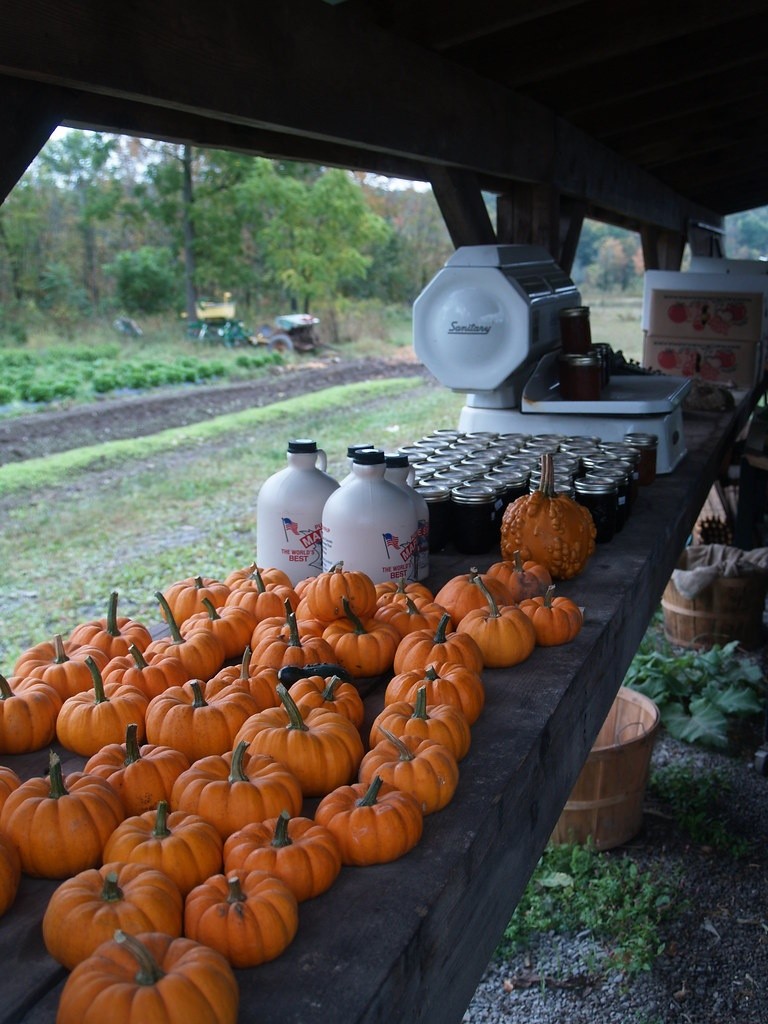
[0,386,768,1024]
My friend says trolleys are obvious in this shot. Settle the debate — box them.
[194,292,344,353]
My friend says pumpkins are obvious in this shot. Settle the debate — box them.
[502,453,597,580]
[0,560,583,1024]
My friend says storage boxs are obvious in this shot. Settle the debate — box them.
[647,288,768,342]
[642,336,763,389]
[757,340,768,388]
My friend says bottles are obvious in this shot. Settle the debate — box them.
[256,438,341,587]
[321,443,431,587]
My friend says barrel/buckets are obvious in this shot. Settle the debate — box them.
[547,685,662,851]
[661,545,767,650]
